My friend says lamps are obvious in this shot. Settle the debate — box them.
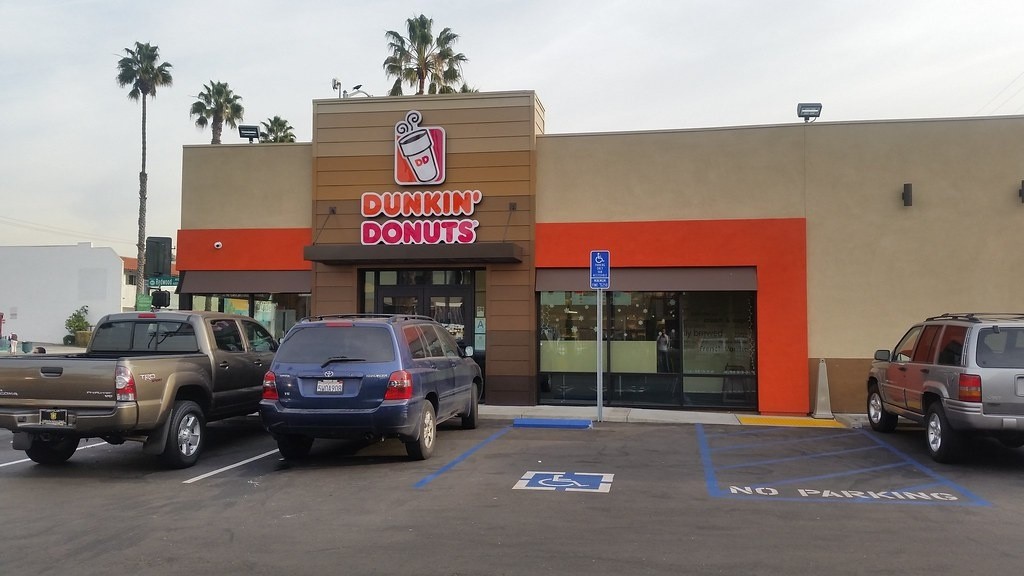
[238,124,261,144]
[1019,180,1024,203]
[902,184,913,207]
[797,103,823,123]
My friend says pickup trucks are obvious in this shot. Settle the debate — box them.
[1,308,280,472]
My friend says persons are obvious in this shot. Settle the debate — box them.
[10,331,18,355]
[657,329,671,373]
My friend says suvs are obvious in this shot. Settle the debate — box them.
[258,312,485,460]
[541,332,553,391]
[697,336,755,368]
[557,335,583,360]
[864,310,1024,462]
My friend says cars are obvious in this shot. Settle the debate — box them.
[669,338,697,361]
